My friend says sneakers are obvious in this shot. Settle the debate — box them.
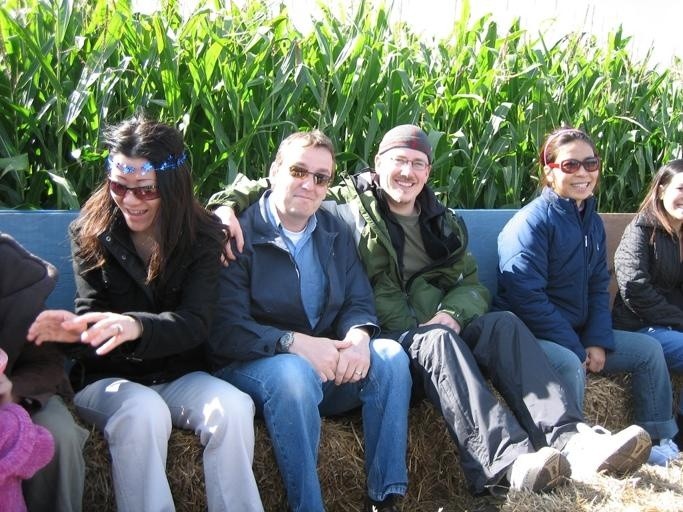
[562,421,653,480]
[646,437,682,466]
[505,445,574,497]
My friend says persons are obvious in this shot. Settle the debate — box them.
[490,126,683,468]
[205,124,652,500]
[25,116,265,512]
[204,128,413,512]
[0,229,91,512]
[611,159,683,452]
[0,348,56,512]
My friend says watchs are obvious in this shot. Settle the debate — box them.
[278,330,294,354]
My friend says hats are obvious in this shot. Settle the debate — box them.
[378,124,436,166]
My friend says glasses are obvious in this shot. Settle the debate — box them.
[287,163,332,186]
[377,155,432,171]
[546,156,601,174]
[108,179,163,201]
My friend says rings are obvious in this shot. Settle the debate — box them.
[115,325,122,335]
[355,372,362,375]
